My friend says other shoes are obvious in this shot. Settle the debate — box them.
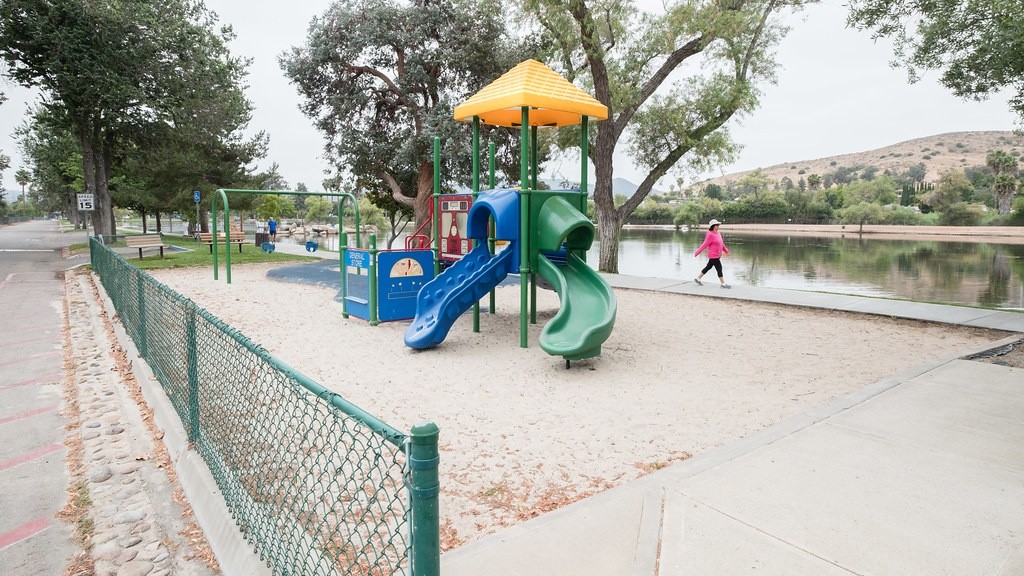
[695,278,703,286]
[721,283,732,289]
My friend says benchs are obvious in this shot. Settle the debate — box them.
[126,235,164,259]
[180,235,193,241]
[199,232,245,253]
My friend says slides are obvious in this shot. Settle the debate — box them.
[403,245,513,349]
[538,252,617,356]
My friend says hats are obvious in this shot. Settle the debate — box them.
[708,219,721,230]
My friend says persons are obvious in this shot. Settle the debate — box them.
[255,215,279,245]
[694,219,732,289]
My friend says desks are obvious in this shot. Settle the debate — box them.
[191,230,201,240]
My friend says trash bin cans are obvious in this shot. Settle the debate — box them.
[255,232,270,246]
